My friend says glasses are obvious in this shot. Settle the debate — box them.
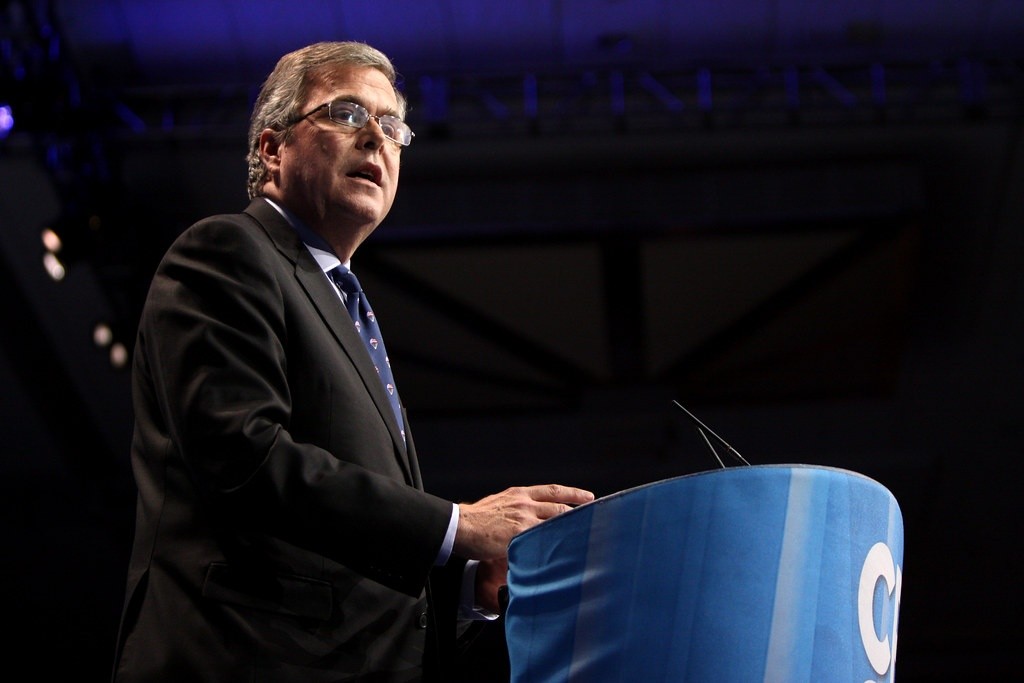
[279,99,415,147]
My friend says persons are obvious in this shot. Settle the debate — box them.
[108,42,595,683]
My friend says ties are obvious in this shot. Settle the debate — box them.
[327,264,408,450]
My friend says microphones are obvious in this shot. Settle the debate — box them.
[671,400,751,467]
[695,427,726,468]
